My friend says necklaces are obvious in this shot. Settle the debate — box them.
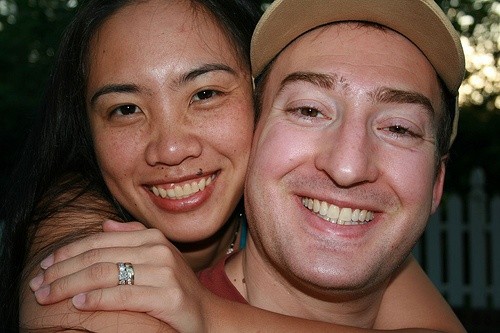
[227,210,242,255]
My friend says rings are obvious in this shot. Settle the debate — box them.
[117,263,136,286]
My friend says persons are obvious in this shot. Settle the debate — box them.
[182,0,465,331]
[10,1,468,333]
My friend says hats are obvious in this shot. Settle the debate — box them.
[247,0,467,153]
